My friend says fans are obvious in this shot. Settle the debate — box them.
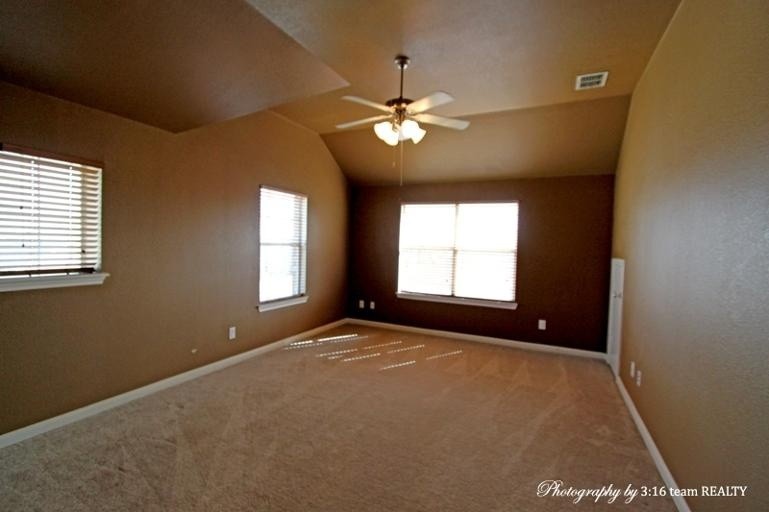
[334,54,470,135]
